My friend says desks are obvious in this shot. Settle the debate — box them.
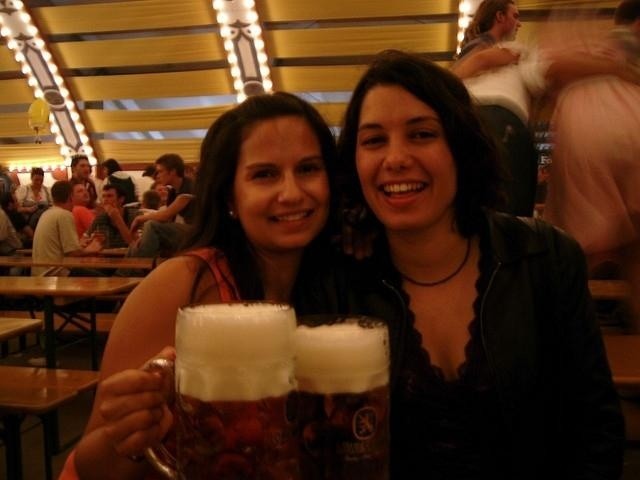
[3,250,157,271]
[534,170,639,458]
[0,364,106,477]
[1,274,147,363]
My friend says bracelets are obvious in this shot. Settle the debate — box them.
[125,457,150,463]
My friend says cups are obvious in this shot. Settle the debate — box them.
[136,298,300,480]
[286,316,392,480]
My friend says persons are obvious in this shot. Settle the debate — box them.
[0,147,194,311]
[446,0,640,285]
[297,51,625,477]
[56,90,376,480]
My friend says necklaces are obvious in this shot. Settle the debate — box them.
[399,235,471,285]
